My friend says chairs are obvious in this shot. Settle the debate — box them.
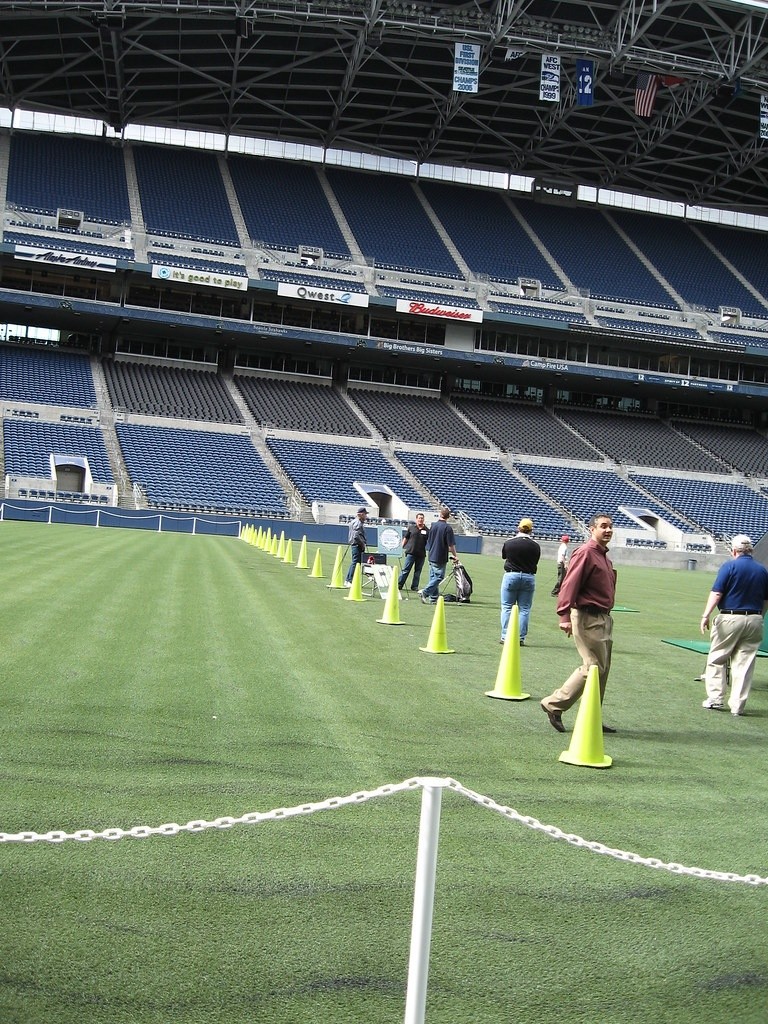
[0,127,768,552]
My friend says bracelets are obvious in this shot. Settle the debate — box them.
[702,615,707,617]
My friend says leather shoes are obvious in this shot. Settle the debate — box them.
[603,723,616,733]
[542,705,565,732]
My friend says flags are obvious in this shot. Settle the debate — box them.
[633,72,687,119]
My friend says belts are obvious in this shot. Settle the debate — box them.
[721,611,761,614]
[507,570,535,575]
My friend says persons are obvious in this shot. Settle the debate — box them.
[343,507,369,586]
[416,507,458,604]
[397,513,430,590]
[701,536,768,718]
[537,511,617,734]
[550,536,570,597]
[500,518,541,647]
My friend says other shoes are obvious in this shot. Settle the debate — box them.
[733,713,740,716]
[417,590,426,603]
[702,699,723,708]
[344,581,351,587]
[412,587,419,591]
[500,641,504,644]
[520,641,523,646]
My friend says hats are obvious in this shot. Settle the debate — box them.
[732,534,752,547]
[562,535,569,542]
[519,518,532,530]
[357,507,369,514]
